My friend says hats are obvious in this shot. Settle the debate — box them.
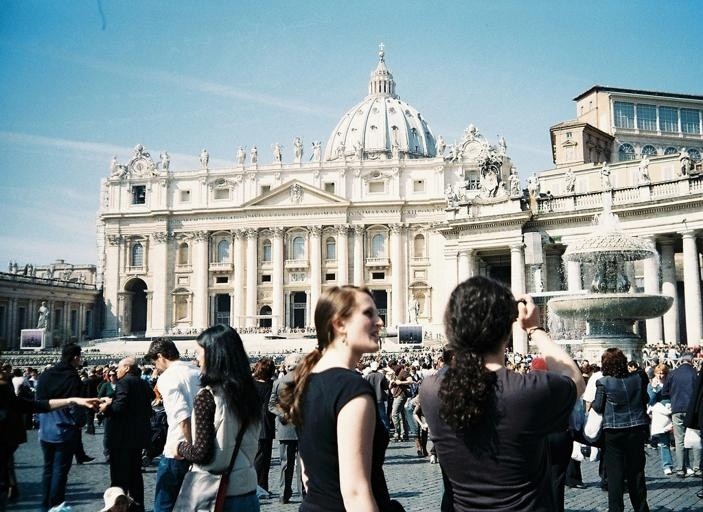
[101,487,134,512]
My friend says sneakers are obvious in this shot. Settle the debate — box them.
[664,468,702,478]
[390,433,440,464]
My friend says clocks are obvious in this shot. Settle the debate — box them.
[461,141,482,159]
[128,157,150,175]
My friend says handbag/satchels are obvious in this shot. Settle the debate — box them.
[582,407,603,442]
[171,465,228,512]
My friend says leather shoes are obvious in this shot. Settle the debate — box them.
[279,496,288,505]
[573,481,587,488]
[77,454,95,465]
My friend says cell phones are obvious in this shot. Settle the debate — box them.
[516,300,527,319]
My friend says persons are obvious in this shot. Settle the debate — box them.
[280,284,390,512]
[444,147,689,199]
[418,274,586,512]
[355,347,455,464]
[250,352,307,503]
[0,324,267,512]
[563,348,703,512]
[504,347,545,375]
[110,135,446,176]
